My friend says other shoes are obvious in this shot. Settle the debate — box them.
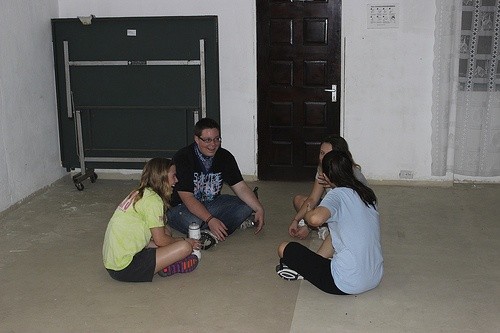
[196,229,218,250]
[237,212,259,231]
[276,263,304,280]
[158,254,198,277]
[317,227,329,240]
[298,218,306,227]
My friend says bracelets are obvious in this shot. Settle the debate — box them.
[206,216,213,223]
[293,219,298,223]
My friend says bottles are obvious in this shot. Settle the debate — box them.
[460,36,468,54]
[188,221,201,261]
[485,13,493,31]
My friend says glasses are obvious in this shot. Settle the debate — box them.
[198,137,221,142]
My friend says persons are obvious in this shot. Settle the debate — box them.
[276,150,384,295]
[102,158,202,282]
[167,117,264,250]
[288,137,370,241]
